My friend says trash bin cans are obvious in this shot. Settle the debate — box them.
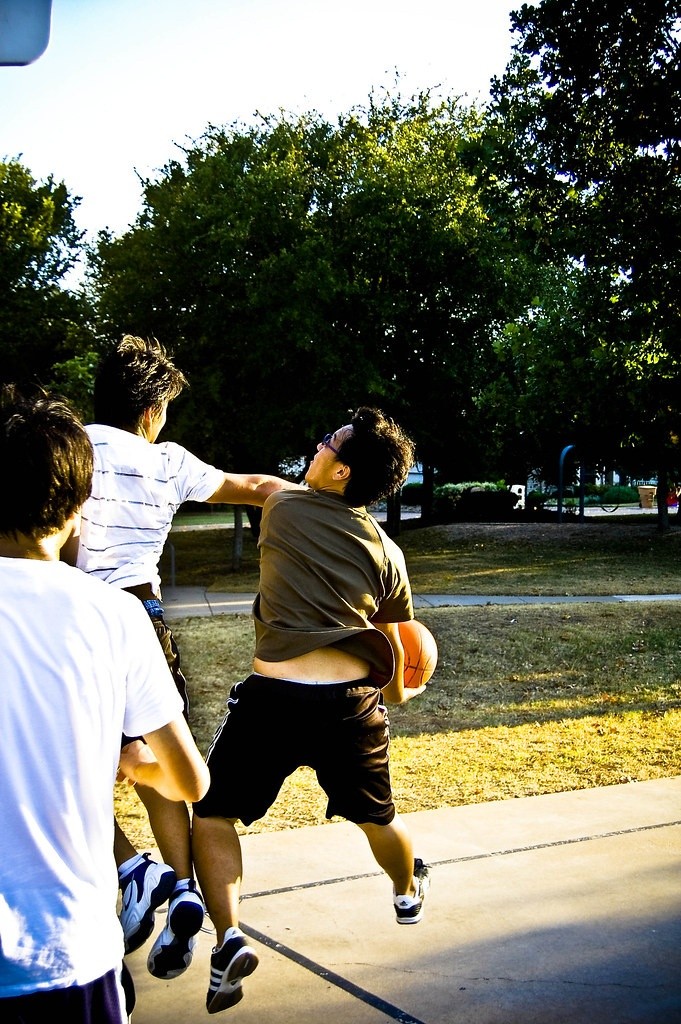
[638,485,659,508]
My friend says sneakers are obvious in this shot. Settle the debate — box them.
[205,927,258,1014]
[147,879,207,980]
[119,852,177,955]
[393,857,431,925]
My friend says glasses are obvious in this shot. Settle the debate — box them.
[323,433,338,456]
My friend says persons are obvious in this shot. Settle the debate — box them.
[0,397,210,1024]
[77,331,311,980]
[189,405,431,1014]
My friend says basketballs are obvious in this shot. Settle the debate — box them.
[397,619,438,688]
